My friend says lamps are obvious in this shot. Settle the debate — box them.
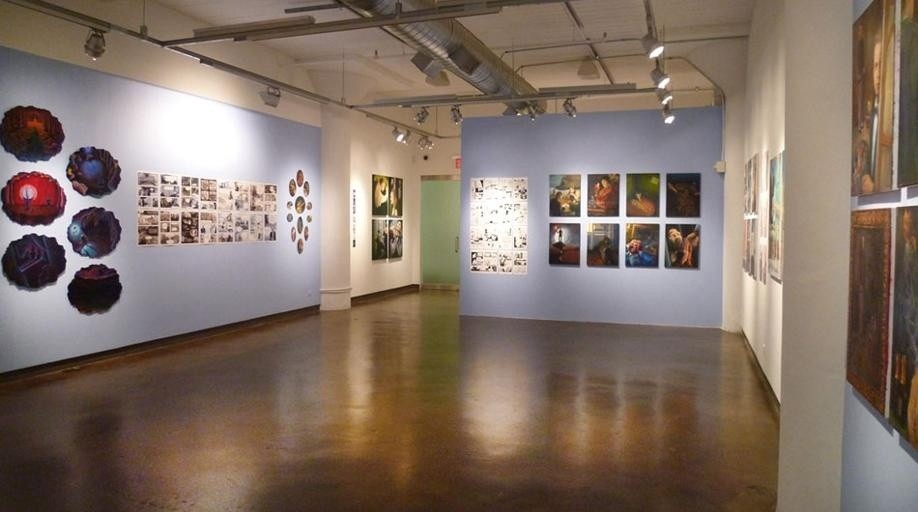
[640,30,665,60]
[390,127,410,146]
[255,86,282,110]
[510,101,547,122]
[81,29,110,62]
[647,66,672,88]
[450,105,466,127]
[422,69,451,86]
[658,104,675,126]
[561,98,580,119]
[576,57,602,84]
[415,134,435,149]
[412,108,431,126]
[653,86,674,108]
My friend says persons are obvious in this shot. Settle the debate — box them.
[667,227,697,268]
[565,188,580,203]
[892,207,917,383]
[595,178,618,211]
[624,239,658,266]
[375,178,389,215]
[548,191,563,217]
[390,220,403,257]
